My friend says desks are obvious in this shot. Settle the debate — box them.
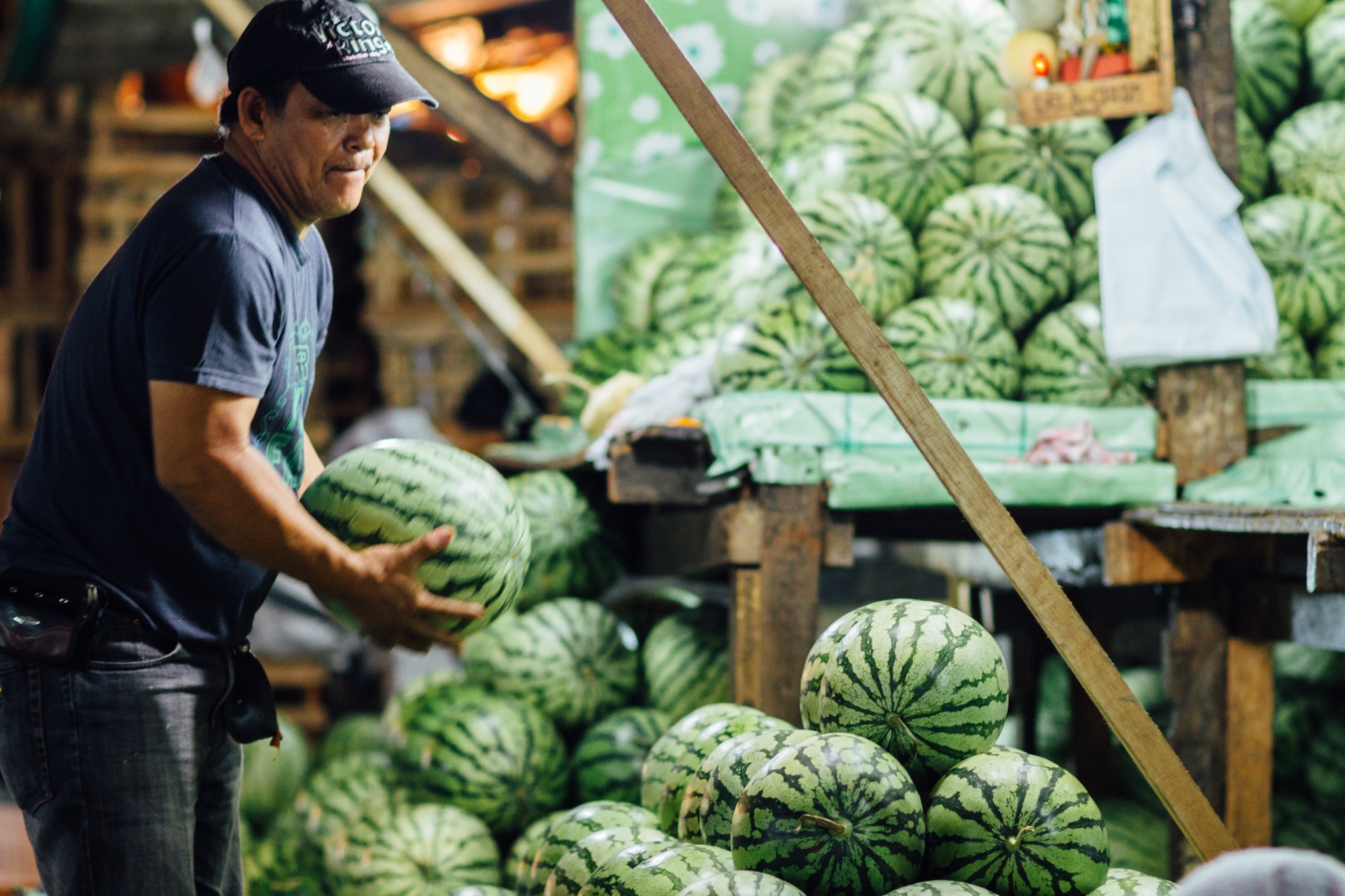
[478,374,1345,861]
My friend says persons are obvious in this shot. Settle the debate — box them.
[0,0,485,895]
[240,363,540,654]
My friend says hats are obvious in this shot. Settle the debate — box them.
[226,0,439,114]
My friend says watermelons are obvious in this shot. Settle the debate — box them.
[241,588,1345,896]
[506,467,617,614]
[563,0,1345,423]
[297,437,526,642]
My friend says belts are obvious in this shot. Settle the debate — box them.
[0,567,147,627]
